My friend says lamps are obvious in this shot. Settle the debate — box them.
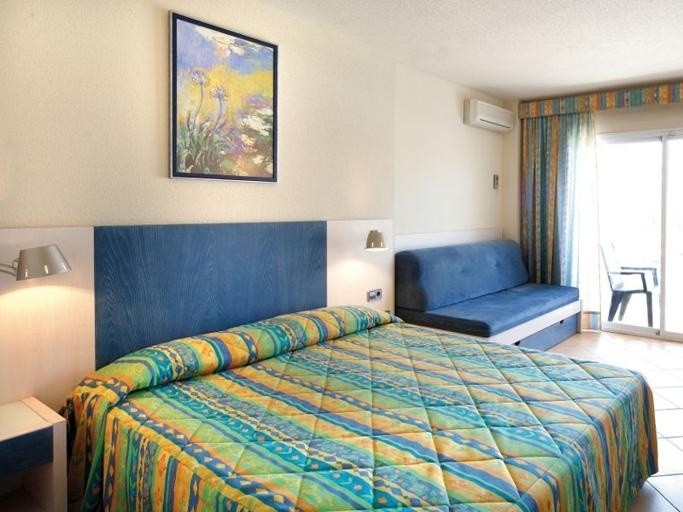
[0,241,70,288]
[364,229,389,250]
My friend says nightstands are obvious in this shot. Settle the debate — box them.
[0,390,75,512]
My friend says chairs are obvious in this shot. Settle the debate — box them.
[595,231,662,331]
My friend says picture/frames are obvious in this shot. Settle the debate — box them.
[169,9,280,184]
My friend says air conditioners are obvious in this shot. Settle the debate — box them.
[464,96,514,134]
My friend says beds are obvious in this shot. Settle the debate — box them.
[89,220,664,512]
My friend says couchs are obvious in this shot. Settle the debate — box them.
[394,234,585,358]
